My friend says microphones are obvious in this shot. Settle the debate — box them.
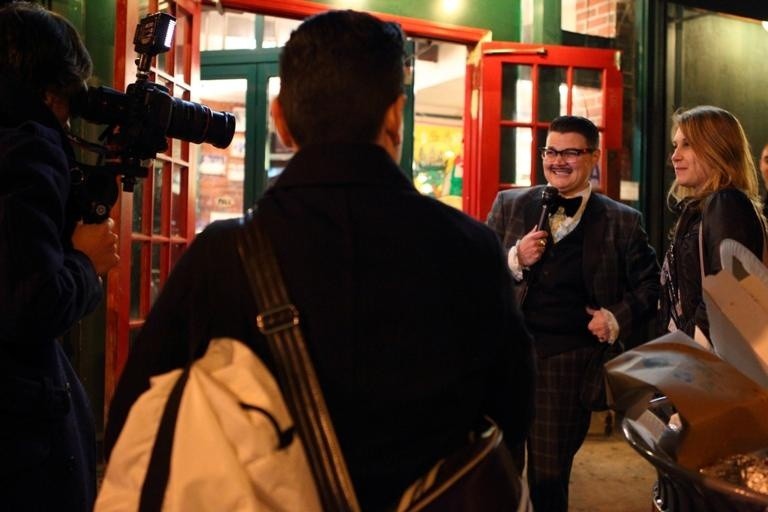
[536,185,559,232]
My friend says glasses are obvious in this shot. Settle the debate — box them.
[539,145,592,164]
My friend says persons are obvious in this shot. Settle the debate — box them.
[657,105,768,354]
[483,115,660,512]
[759,141,768,219]
[104,7,539,512]
[1,2,120,512]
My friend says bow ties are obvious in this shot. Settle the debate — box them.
[547,194,581,218]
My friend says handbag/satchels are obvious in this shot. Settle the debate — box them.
[90,218,325,511]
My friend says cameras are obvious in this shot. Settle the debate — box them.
[90,81,237,162]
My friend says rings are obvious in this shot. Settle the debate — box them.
[538,238,546,246]
